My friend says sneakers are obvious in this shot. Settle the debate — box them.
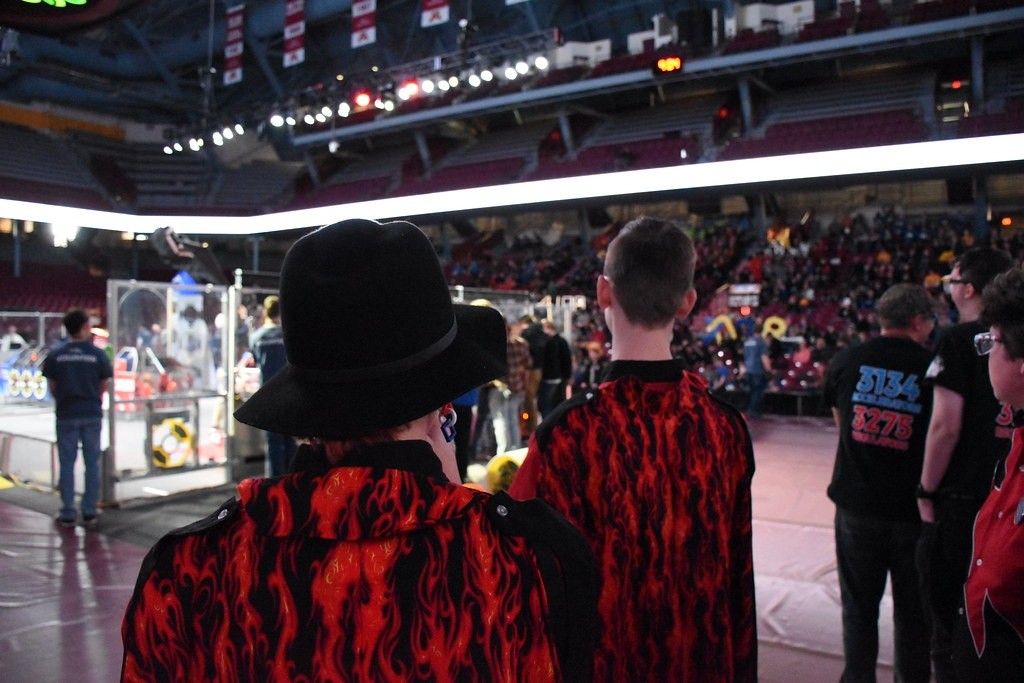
[82,507,106,525]
[54,517,76,531]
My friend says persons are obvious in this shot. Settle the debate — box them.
[821,245,1024,683]
[761,207,1024,392]
[42,310,113,527]
[120,215,757,683]
[1,325,28,351]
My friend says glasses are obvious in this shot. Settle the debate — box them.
[974,332,1006,357]
[941,273,963,294]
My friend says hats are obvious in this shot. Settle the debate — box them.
[234,219,509,437]
